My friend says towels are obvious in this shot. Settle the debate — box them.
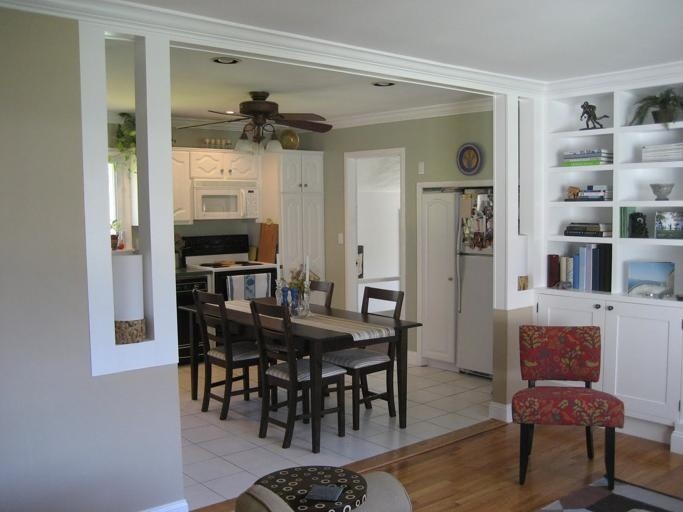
[226,273,271,302]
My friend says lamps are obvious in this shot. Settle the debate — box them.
[235,123,283,153]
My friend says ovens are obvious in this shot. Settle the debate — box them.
[209,268,279,338]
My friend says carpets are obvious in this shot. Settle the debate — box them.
[531,474,683,512]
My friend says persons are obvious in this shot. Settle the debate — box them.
[580,101,610,129]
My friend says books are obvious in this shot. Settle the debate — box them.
[546,148,613,292]
[641,141,683,163]
[619,206,636,238]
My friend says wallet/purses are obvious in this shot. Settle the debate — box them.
[305,484,343,501]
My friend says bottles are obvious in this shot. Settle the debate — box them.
[117,231,125,249]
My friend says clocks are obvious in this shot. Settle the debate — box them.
[456,142,481,176]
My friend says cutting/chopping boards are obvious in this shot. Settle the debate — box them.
[256,223,279,263]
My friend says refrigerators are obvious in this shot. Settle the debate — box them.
[455,191,495,379]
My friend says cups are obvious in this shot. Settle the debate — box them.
[111,235,119,252]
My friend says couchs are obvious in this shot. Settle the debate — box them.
[230,466,413,512]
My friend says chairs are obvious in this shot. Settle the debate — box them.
[320,286,405,430]
[191,289,279,419]
[512,325,625,490]
[249,301,347,446]
[256,280,334,412]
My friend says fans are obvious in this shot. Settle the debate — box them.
[175,92,333,134]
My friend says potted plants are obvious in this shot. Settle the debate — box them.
[629,89,683,127]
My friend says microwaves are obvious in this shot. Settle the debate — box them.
[194,187,257,223]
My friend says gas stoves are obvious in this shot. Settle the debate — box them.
[197,261,278,272]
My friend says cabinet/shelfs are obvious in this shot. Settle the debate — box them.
[539,82,683,305]
[536,288,683,455]
[261,149,326,194]
[172,147,193,222]
[176,275,209,366]
[420,188,460,375]
[266,195,324,298]
[189,140,259,180]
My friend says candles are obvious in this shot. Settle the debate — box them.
[277,254,280,284]
[305,256,309,282]
